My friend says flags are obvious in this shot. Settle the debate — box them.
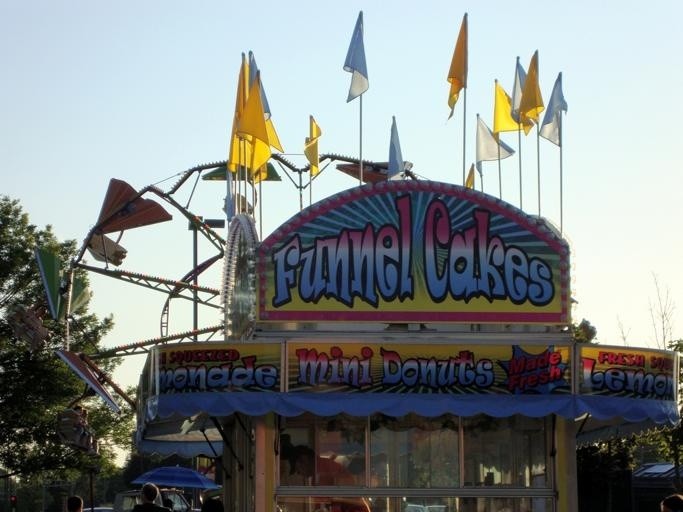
[343,10,370,104]
[231,51,284,184]
[304,116,322,176]
[465,114,515,187]
[387,116,406,181]
[494,52,566,147]
[447,13,466,120]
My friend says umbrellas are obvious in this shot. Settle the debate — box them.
[129,465,221,491]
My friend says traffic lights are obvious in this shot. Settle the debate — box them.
[9,494,17,505]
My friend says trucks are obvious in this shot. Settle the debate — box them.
[83,488,200,512]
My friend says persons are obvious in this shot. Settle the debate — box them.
[294,445,370,511]
[63,492,85,511]
[660,493,683,512]
[131,482,169,511]
[162,497,175,511]
[200,497,225,511]
